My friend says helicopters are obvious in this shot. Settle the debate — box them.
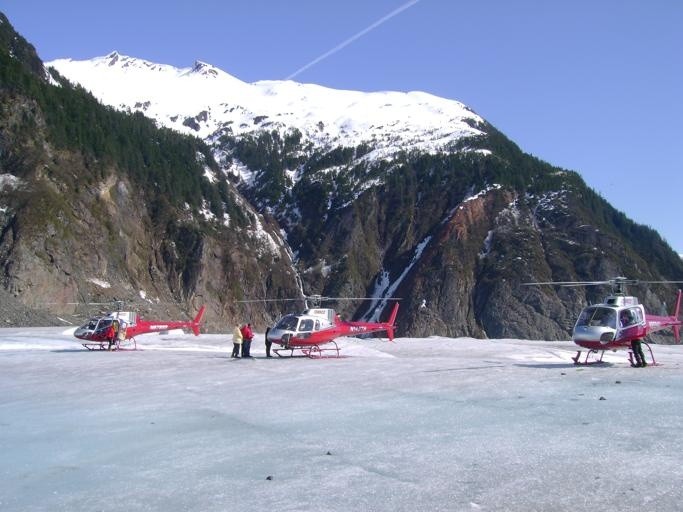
[39,293,209,353]
[518,275,682,371]
[231,291,405,362]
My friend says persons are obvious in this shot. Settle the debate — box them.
[104,323,115,351]
[247,323,255,358]
[264,326,273,358]
[239,323,252,358]
[625,315,647,368]
[231,323,244,359]
